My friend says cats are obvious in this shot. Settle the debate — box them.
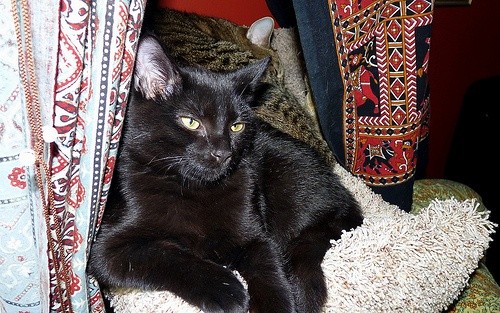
[89,36,364,313]
[143,4,337,168]
